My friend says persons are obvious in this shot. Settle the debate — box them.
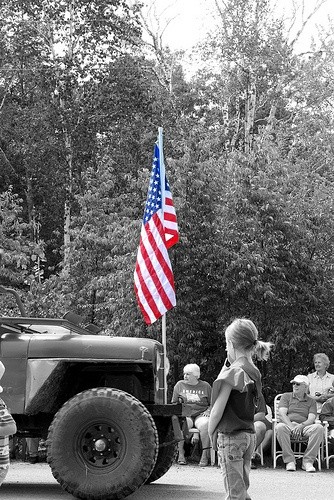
[208,318,273,500]
[0,361,17,485]
[250,406,273,468]
[275,375,323,471]
[307,353,334,468]
[172,363,211,466]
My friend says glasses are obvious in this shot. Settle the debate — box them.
[293,383,301,386]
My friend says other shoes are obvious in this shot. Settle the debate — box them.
[200,455,208,467]
[178,456,187,465]
[250,457,258,468]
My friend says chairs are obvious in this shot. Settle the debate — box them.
[250,405,272,466]
[317,408,334,470]
[272,394,322,471]
[188,427,216,465]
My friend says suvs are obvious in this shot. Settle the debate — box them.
[0,285,193,500]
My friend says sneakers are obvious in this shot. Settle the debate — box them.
[302,463,315,472]
[286,462,296,471]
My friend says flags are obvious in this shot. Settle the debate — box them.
[134,140,181,325]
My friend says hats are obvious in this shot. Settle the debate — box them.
[289,375,310,387]
